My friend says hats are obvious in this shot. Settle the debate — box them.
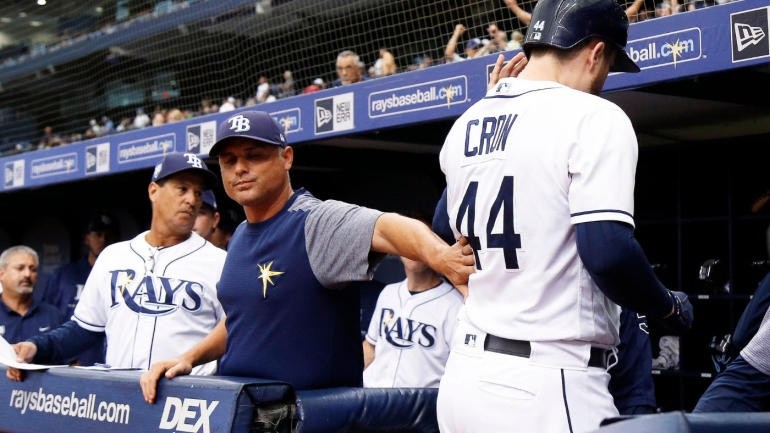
[523,0,640,73]
[467,38,483,49]
[209,110,287,157]
[202,190,218,211]
[153,153,214,192]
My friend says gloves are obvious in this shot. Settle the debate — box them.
[664,290,695,333]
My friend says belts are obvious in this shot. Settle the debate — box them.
[460,333,615,368]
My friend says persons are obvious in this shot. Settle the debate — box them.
[41,212,118,364]
[428,1,696,433]
[692,270,770,412]
[192,189,232,251]
[0,245,70,375]
[363,256,465,389]
[5,152,228,400]
[603,306,680,416]
[0,0,713,152]
[138,110,475,405]
